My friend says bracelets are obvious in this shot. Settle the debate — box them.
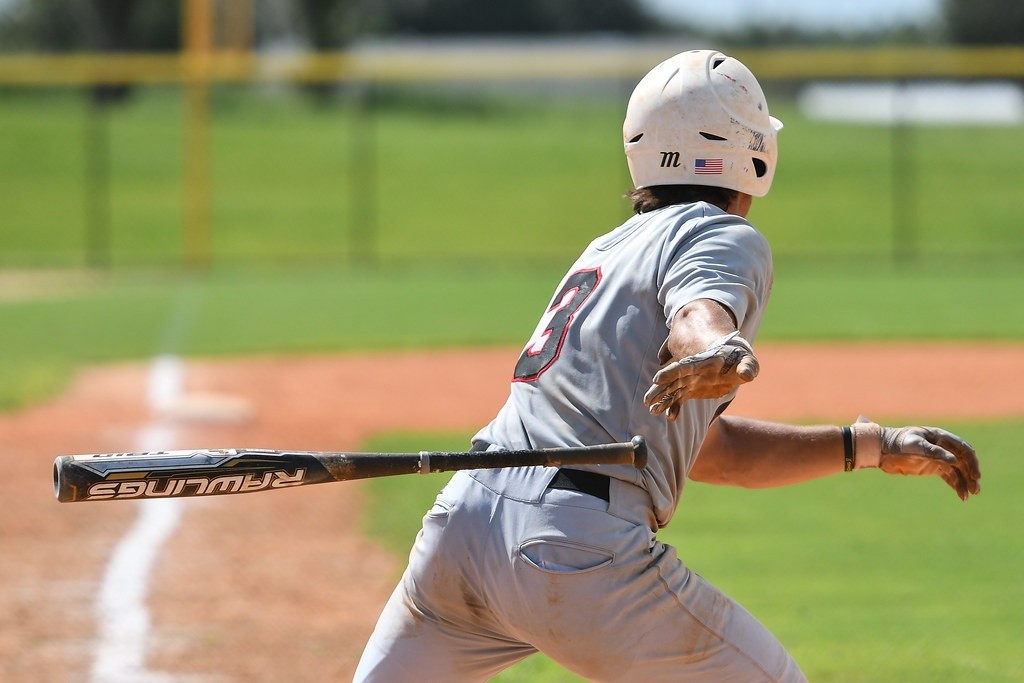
[852,423,881,470]
[842,426,853,472]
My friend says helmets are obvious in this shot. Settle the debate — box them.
[622,49,783,197]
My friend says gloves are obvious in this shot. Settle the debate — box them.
[853,422,981,503]
[642,330,760,421]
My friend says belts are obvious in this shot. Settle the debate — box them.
[466,439,611,505]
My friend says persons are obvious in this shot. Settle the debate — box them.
[351,50,983,683]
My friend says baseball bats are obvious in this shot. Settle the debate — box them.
[50,427,651,515]
